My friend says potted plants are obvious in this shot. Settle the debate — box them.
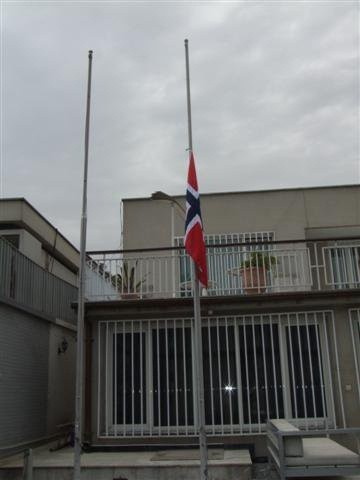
[237,250,276,292]
[111,258,150,301]
